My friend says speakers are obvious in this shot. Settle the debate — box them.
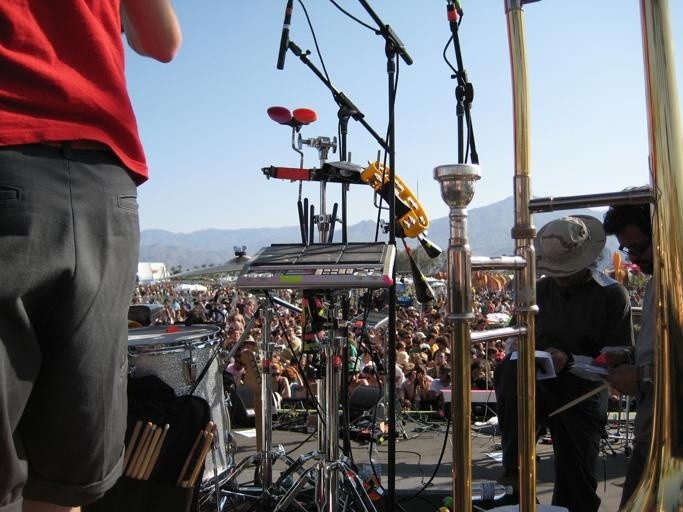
[342,385,385,426]
[229,385,278,422]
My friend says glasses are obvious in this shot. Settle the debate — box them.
[618,240,651,258]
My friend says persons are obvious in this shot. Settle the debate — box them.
[0,0,183,512]
[126,259,653,450]
[590,184,659,511]
[491,213,635,511]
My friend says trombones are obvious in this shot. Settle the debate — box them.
[434,0,683,512]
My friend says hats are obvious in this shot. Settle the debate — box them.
[533,214,606,278]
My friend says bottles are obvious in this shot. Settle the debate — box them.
[362,442,383,501]
[469,479,515,501]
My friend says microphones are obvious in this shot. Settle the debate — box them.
[277,0,294,69]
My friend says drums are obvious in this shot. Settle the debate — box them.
[128,324,237,493]
